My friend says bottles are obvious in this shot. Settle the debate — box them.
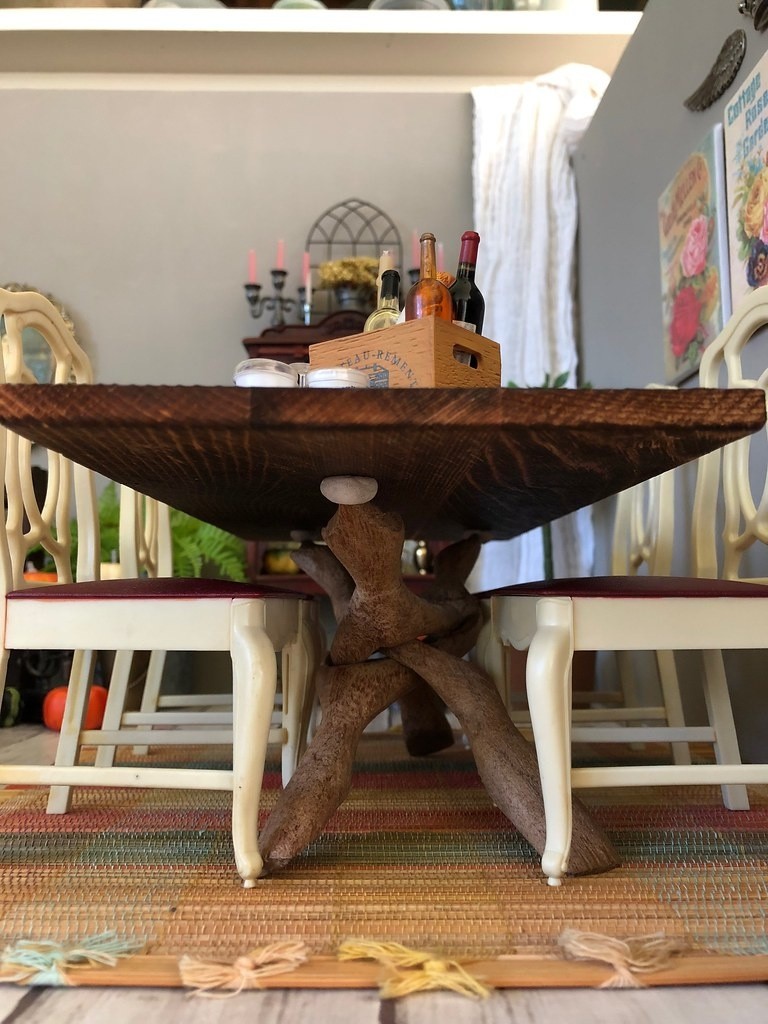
[449,231,485,370]
[363,270,402,331]
[405,233,452,323]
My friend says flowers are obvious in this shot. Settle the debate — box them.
[317,256,379,289]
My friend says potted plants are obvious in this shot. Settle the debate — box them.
[23,480,284,710]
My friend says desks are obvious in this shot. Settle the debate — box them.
[0,383,767,874]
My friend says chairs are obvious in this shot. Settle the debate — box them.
[468,282,768,890]
[0,288,318,890]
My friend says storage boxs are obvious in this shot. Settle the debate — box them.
[310,315,500,389]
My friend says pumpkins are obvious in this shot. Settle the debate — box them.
[42,683,110,731]
[21,560,58,583]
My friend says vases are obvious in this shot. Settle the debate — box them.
[334,283,372,312]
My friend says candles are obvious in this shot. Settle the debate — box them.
[276,239,284,270]
[303,253,310,288]
[249,249,257,283]
